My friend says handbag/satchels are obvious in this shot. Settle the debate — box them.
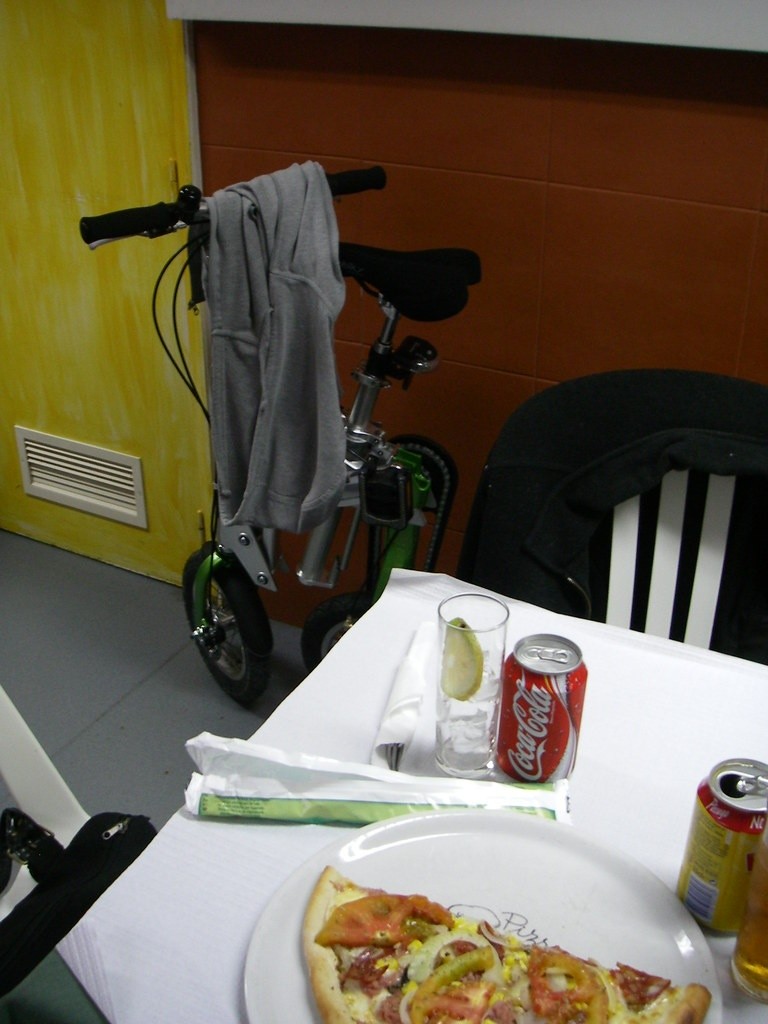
[0,806,158,999]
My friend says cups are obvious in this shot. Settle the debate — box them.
[435,593,509,779]
[730,815,768,1004]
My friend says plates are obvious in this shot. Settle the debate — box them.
[243,808,722,1024]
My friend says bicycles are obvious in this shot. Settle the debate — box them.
[79,160,480,701]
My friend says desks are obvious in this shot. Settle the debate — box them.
[0,569,768,1024]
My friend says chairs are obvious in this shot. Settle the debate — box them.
[0,684,90,923]
[480,369,768,664]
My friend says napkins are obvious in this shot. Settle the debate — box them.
[370,620,439,771]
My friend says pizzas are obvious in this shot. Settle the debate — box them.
[301,865,712,1024]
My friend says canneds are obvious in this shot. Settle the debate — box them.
[495,633,588,782]
[676,758,768,938]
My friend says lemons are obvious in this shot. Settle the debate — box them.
[439,617,484,701]
